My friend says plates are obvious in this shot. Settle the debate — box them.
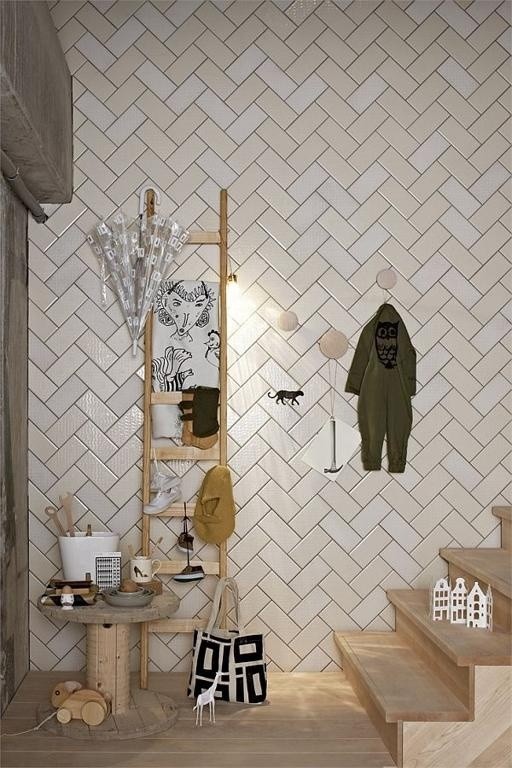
[103,589,155,606]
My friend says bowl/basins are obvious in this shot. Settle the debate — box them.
[116,587,144,596]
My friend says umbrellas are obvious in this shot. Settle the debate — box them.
[86,187,190,356]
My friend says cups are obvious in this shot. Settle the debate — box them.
[130,556,160,583]
[61,594,74,610]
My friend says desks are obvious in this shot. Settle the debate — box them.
[35,591,179,741]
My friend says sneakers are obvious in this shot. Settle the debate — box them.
[141,487,182,516]
[177,531,195,553]
[149,471,182,493]
[172,565,206,583]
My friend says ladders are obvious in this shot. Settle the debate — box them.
[139,189,230,694]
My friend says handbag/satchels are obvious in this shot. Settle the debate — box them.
[186,626,272,706]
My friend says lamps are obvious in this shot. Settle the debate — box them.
[225,239,245,319]
